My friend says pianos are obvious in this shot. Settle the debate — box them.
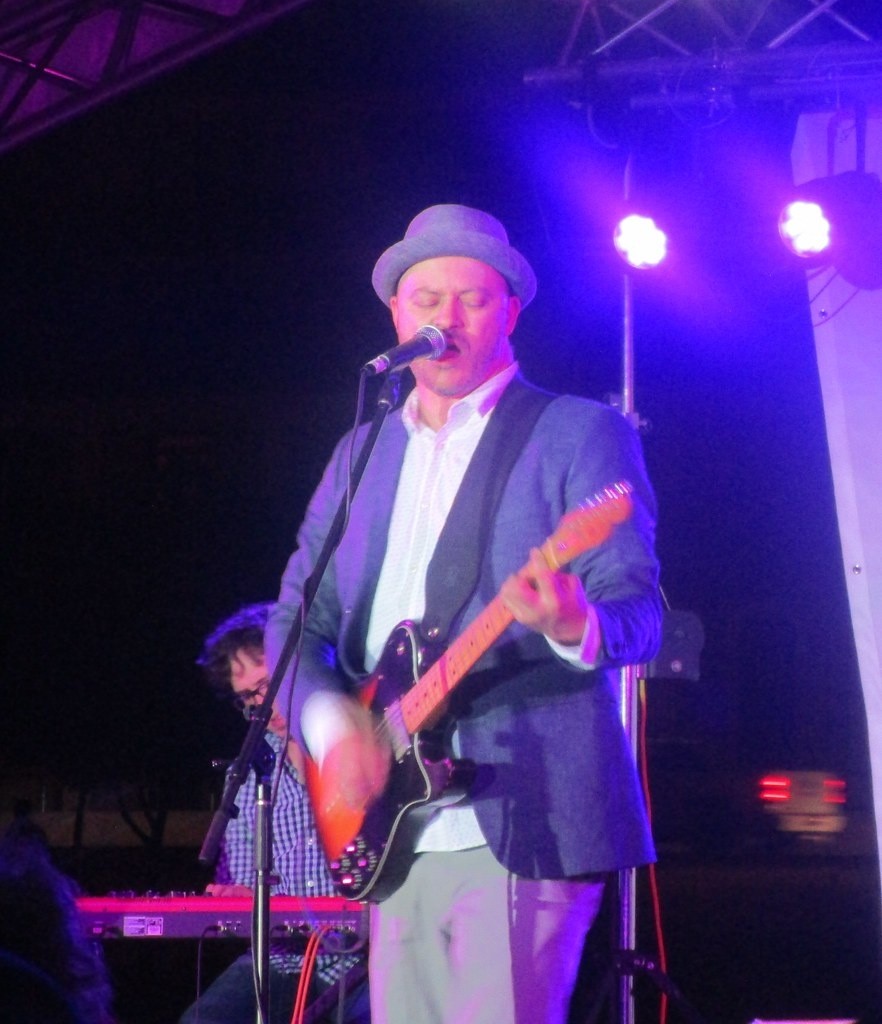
[68,890,369,942]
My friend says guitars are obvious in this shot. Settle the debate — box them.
[304,481,636,901]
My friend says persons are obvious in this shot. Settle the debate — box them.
[264,203,663,1023]
[0,844,110,1024]
[184,606,371,1024]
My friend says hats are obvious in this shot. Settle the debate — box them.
[371,205,537,308]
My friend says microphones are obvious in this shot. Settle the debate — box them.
[359,324,447,378]
